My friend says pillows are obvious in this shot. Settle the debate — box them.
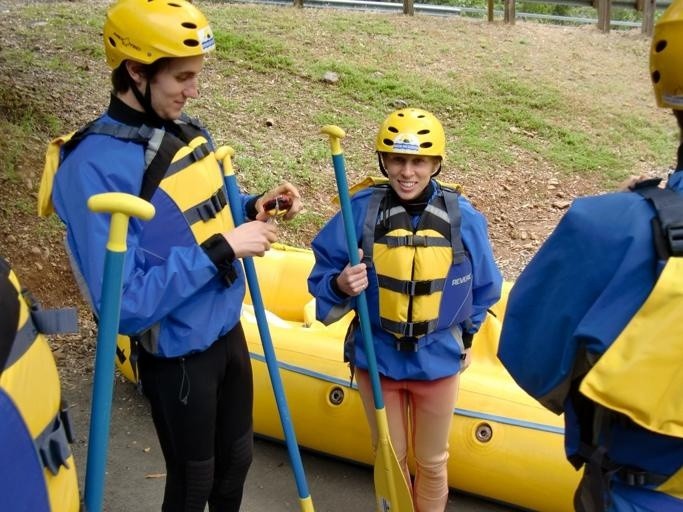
[376,108,446,162]
[649,1,682,111]
[101,1,215,71]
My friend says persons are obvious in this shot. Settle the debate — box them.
[307,106,503,512]
[51,0,303,512]
[1,258,80,512]
[495,4,683,512]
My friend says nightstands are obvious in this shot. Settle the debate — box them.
[320,124,415,512]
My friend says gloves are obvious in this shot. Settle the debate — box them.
[111,237,585,511]
